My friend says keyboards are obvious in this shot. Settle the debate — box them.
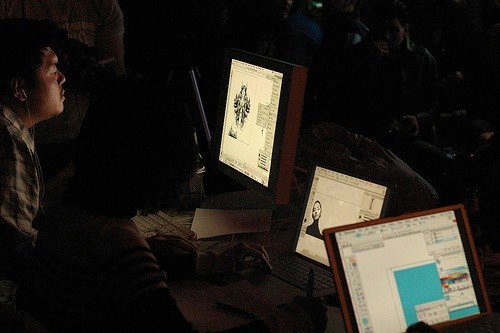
[129,209,198,241]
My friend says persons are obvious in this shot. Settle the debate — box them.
[0,18,197,333]
[304,201,324,240]
[243,0,500,268]
[16,74,328,333]
[0,0,127,176]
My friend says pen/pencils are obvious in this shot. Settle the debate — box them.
[307,269,314,298]
[214,300,259,319]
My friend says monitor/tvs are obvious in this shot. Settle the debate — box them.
[201,47,306,213]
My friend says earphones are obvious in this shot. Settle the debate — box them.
[18,88,27,99]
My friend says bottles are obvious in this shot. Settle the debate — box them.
[463,188,486,265]
[188,128,207,171]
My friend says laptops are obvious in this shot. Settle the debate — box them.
[233,161,397,307]
[323,205,500,333]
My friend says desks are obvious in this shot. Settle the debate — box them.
[164,188,346,333]
